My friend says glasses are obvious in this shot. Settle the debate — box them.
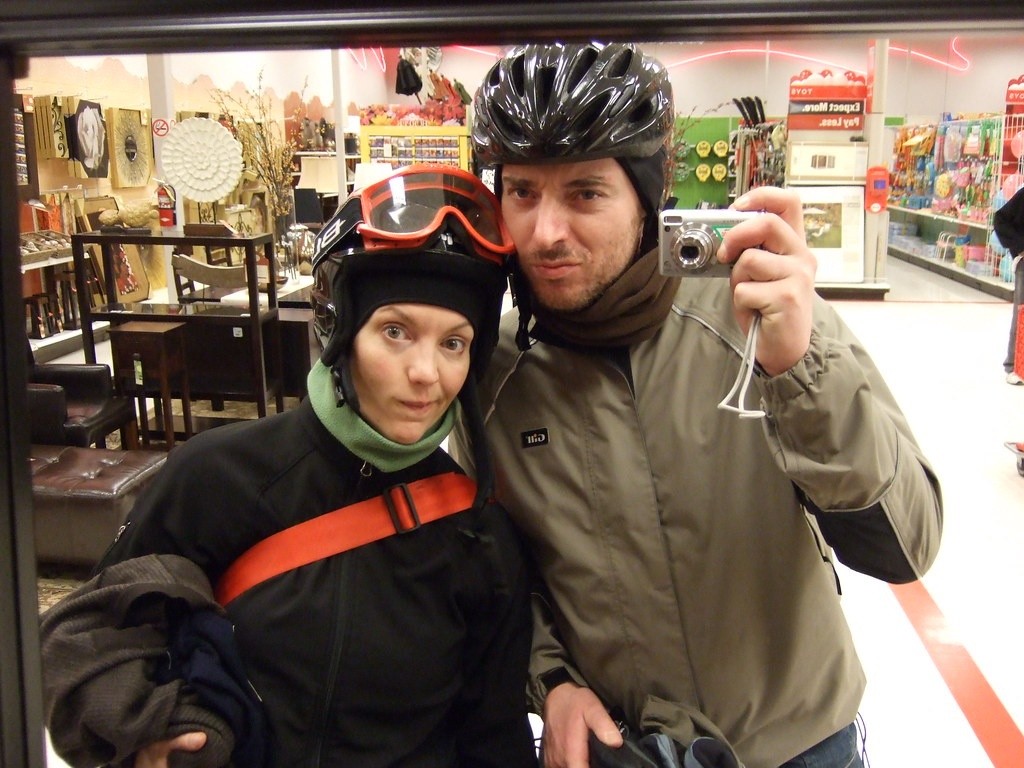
[347,166,518,257]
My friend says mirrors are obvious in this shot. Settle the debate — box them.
[0,0,1024,768]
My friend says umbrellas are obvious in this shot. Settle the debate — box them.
[396,55,473,106]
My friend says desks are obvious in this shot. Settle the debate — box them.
[178,255,314,310]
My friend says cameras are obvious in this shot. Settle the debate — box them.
[659,209,767,278]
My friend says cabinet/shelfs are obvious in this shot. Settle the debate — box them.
[72,227,284,452]
[883,109,1005,277]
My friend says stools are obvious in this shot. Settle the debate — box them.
[29,443,168,565]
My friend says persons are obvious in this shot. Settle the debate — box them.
[449,42,944,768]
[993,187,1024,385]
[38,164,539,768]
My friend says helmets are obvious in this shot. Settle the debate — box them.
[310,166,508,367]
[470,40,676,249]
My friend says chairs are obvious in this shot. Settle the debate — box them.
[293,188,325,237]
[172,250,199,304]
[205,245,232,269]
[25,332,139,449]
